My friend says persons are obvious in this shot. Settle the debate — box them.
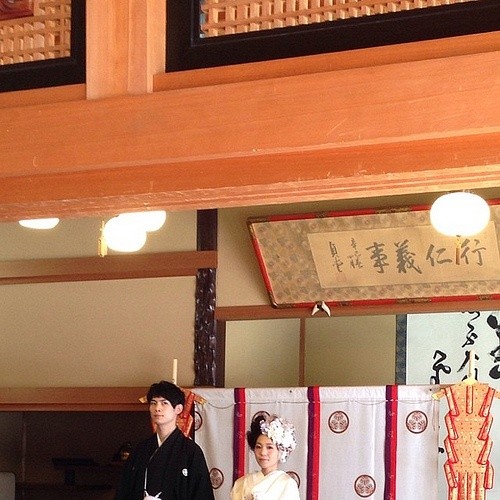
[115,380,215,500]
[229,415,300,500]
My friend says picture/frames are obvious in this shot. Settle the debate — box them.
[246,198,500,310]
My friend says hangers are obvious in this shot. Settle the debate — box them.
[139,377,207,406]
[432,373,500,400]
